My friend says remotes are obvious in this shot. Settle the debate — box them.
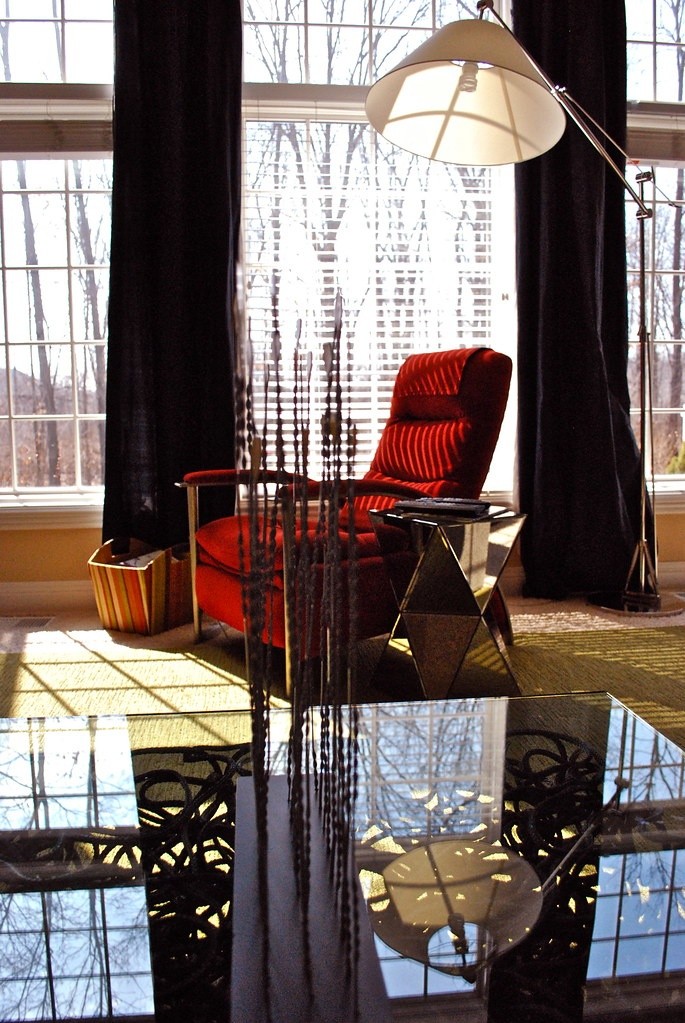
[394,497,492,518]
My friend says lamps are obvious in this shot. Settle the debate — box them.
[366,707,629,983]
[365,0,684,617]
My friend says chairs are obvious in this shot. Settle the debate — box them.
[175,349,515,699]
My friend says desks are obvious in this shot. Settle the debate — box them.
[1,692,684,1023]
[363,507,527,699]
